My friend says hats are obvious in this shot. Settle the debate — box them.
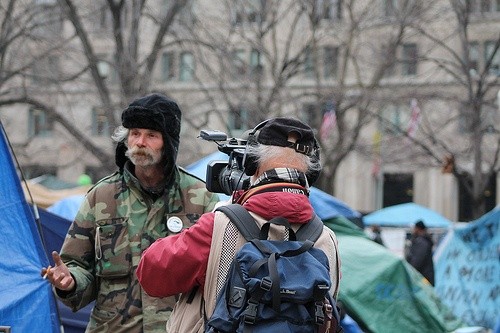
[416,222,425,229]
[115,95,181,189]
[257,117,314,156]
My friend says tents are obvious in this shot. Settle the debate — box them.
[326,219,491,333]
[0,120,66,333]
[19,146,361,333]
[362,202,454,260]
[431,206,500,333]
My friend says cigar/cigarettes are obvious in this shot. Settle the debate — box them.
[42,265,52,280]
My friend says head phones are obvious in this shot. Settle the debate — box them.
[242,118,322,188]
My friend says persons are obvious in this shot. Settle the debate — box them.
[41,93,222,333]
[135,113,344,333]
[404,220,436,287]
[368,224,385,245]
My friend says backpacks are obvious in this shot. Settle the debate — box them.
[205,204,341,333]
